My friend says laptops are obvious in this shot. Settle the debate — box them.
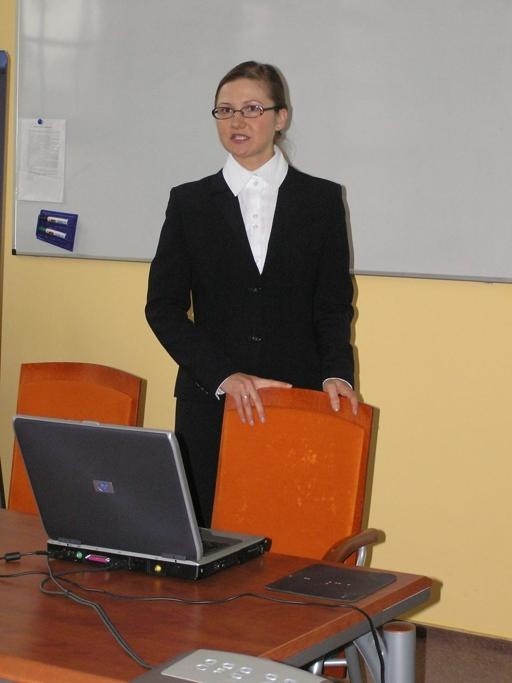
[12,416,272,581]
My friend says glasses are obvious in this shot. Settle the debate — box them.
[211,102,283,118]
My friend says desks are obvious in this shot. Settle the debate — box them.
[1,508,434,682]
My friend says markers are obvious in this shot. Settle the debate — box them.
[38,215,69,240]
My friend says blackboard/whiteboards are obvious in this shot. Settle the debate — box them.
[12,0,512,282]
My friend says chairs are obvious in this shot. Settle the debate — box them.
[7,360,143,517]
[207,387,378,683]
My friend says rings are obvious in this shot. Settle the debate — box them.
[241,394,248,399]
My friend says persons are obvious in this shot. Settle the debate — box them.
[144,60,359,529]
